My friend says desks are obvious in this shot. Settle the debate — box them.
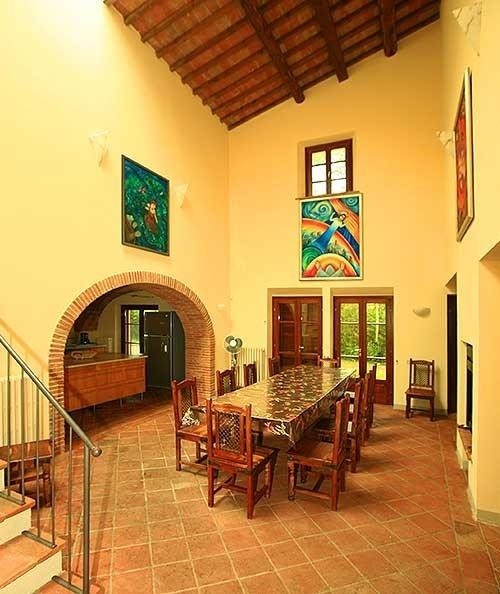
[210,364,358,447]
[0,439,53,507]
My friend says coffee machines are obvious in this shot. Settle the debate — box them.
[80,332,89,345]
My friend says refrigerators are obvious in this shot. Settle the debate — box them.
[144,311,185,390]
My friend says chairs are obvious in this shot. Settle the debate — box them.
[405,358,435,422]
[268,356,282,377]
[350,363,377,428]
[171,376,221,478]
[286,393,351,512]
[215,367,237,396]
[317,355,341,368]
[342,370,375,440]
[243,362,257,387]
[310,378,364,473]
[204,398,280,519]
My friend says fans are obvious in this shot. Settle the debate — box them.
[223,335,243,368]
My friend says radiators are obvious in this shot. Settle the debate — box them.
[233,347,264,387]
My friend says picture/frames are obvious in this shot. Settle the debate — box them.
[299,193,364,281]
[121,154,170,257]
[452,68,475,243]
